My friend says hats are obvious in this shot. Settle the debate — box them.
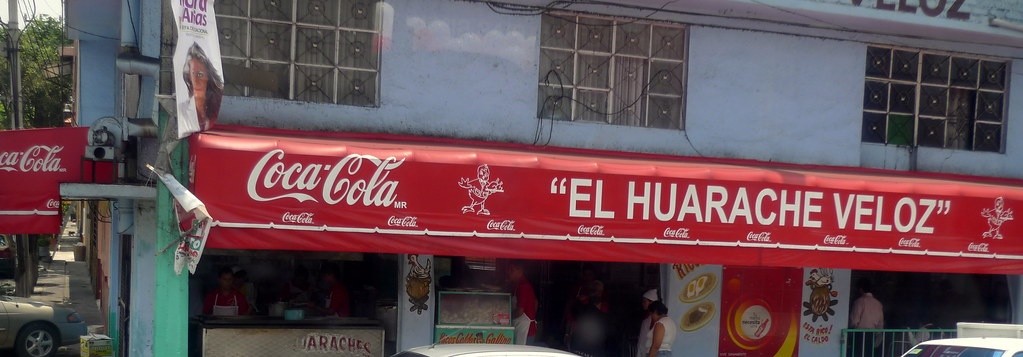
[643,288,658,301]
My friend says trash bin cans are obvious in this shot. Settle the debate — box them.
[73,244,86,261]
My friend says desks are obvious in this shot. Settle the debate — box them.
[196,315,385,357]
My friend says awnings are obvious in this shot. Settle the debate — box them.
[185,124,1023,275]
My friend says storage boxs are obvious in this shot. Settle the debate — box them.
[80,333,114,357]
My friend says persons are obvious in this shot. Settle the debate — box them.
[637,289,658,357]
[562,280,608,357]
[849,278,884,357]
[177,42,224,139]
[505,261,538,345]
[201,268,253,316]
[305,264,348,318]
[644,300,676,357]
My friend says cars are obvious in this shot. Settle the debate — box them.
[900,338,1023,357]
[391,342,580,357]
[0,234,17,278]
[0,294,87,357]
